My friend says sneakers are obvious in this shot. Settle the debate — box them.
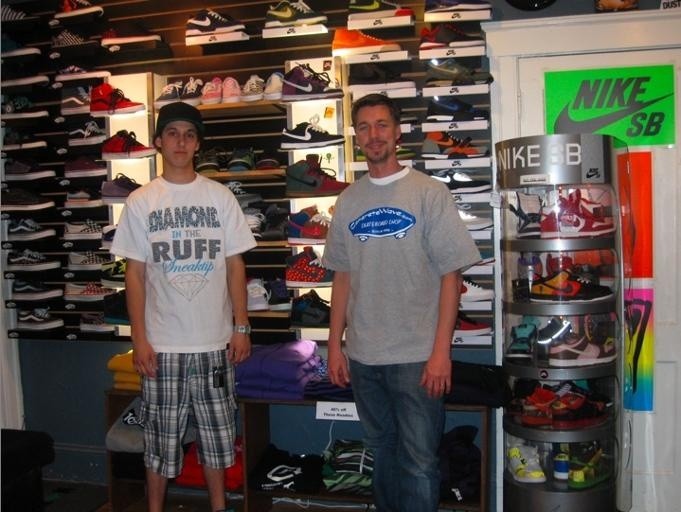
[512,253,614,305]
[197,119,349,201]
[153,64,345,110]
[510,377,614,432]
[246,246,335,328]
[332,0,492,158]
[505,315,618,368]
[507,188,617,240]
[429,168,492,194]
[507,435,612,489]
[456,205,493,231]
[454,317,491,338]
[2,1,161,331]
[185,9,246,36]
[240,203,332,245]
[459,279,495,302]
[265,1,328,28]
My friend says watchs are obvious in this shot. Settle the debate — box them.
[234,325,252,333]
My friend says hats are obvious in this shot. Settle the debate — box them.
[153,102,205,154]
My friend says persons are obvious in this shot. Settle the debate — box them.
[109,103,258,511]
[320,92,484,512]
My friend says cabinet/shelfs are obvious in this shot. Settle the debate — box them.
[103,380,494,512]
[2,0,494,345]
[495,130,635,512]
